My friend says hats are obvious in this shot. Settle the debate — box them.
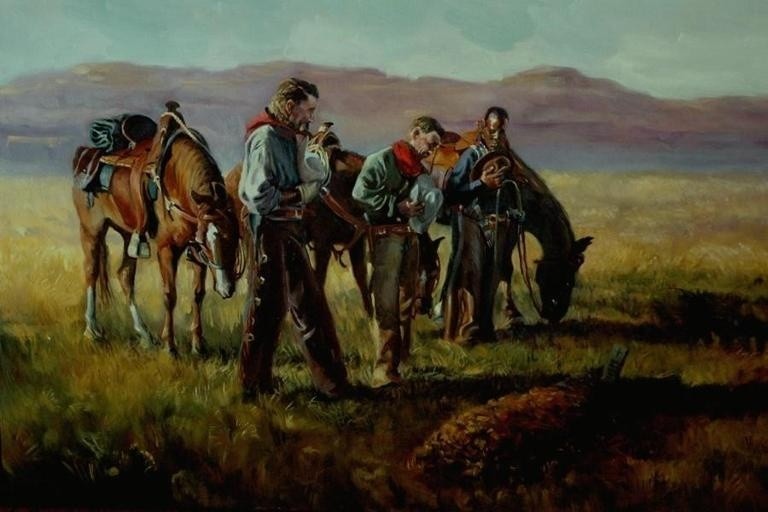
[408,174,444,235]
[470,150,515,192]
[295,132,332,188]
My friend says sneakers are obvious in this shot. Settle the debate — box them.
[241,376,283,404]
[318,383,368,401]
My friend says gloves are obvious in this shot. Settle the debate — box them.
[295,180,321,206]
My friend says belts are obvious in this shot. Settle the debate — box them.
[277,209,304,220]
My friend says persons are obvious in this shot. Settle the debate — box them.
[450,104,526,344]
[237,76,351,399]
[351,115,445,388]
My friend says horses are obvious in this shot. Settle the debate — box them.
[70,126,241,361]
